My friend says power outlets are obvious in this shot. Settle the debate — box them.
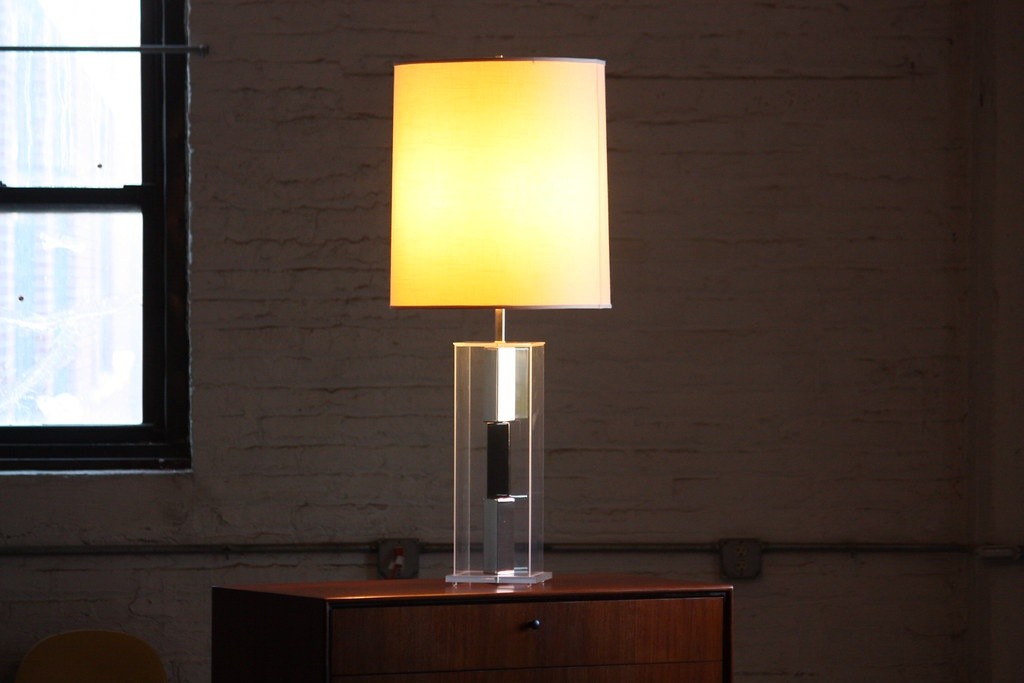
[376,537,418,580]
[717,537,763,583]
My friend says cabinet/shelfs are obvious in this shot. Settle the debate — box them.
[210,575,735,682]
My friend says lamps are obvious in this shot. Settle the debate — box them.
[388,57,613,585]
